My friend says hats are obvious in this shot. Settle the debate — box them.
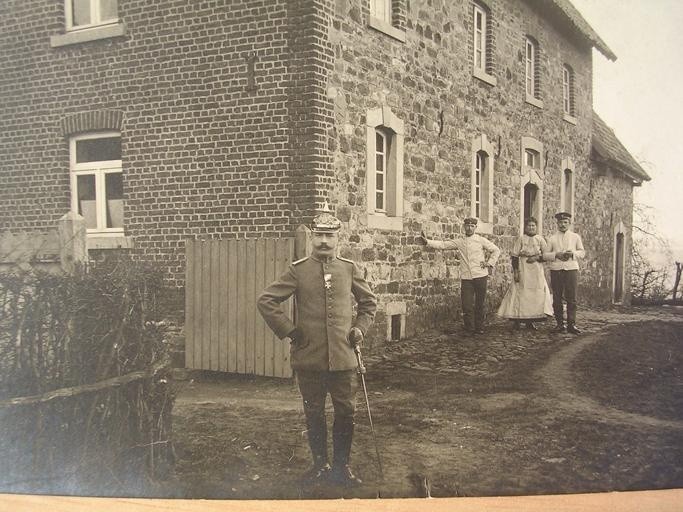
[555,212,573,220]
[464,217,478,225]
[309,211,342,234]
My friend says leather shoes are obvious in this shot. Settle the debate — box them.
[510,319,584,337]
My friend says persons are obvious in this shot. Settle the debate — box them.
[497,218,553,328]
[256,213,377,487]
[420,217,501,336]
[541,212,586,334]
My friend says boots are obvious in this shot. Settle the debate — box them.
[299,413,363,487]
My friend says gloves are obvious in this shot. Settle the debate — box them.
[349,327,364,347]
[287,326,311,353]
